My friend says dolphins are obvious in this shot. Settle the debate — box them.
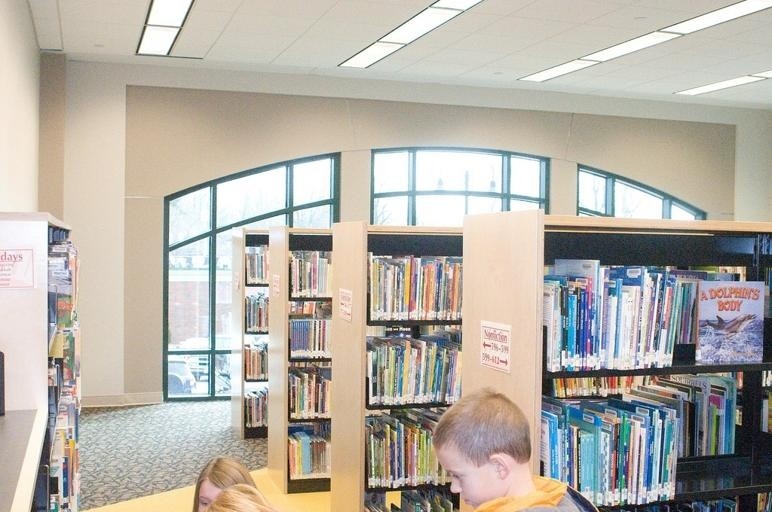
[701,312,758,340]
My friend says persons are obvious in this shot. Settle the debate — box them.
[191,456,278,512]
[433,386,598,512]
[202,484,283,512]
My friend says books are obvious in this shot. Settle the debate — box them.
[245,247,268,427]
[543,257,771,511]
[47,223,82,512]
[368,253,463,511]
[290,251,331,477]
